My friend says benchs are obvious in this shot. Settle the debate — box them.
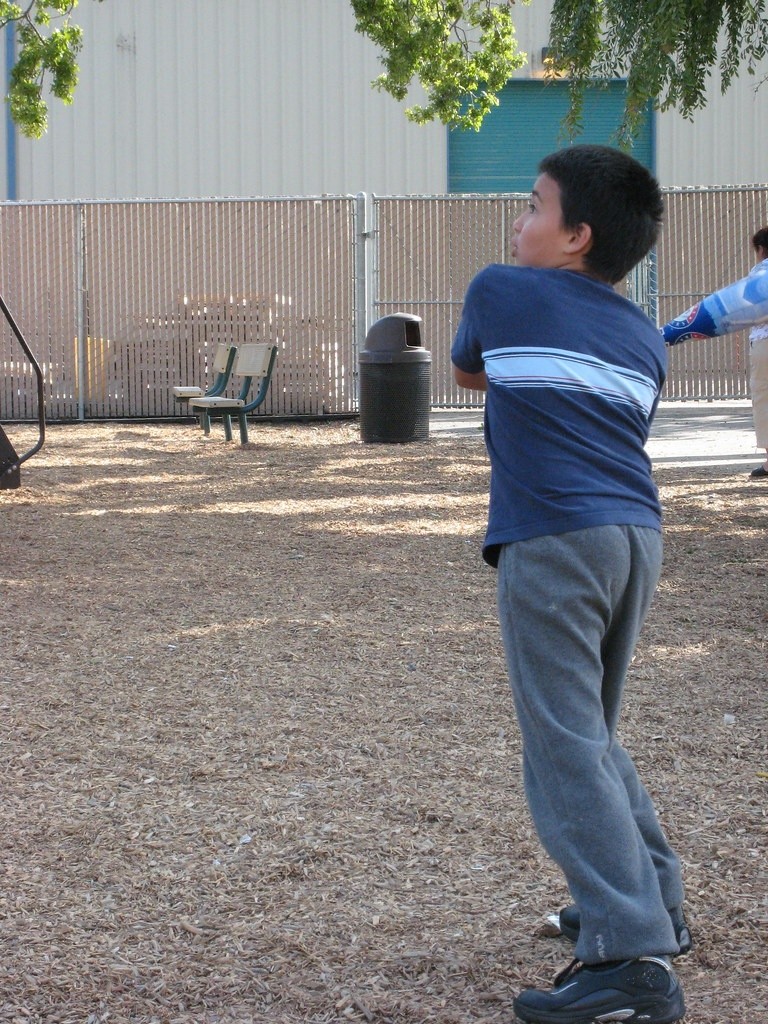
[189,343,278,444]
[173,343,238,435]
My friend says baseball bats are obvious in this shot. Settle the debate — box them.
[659,267,768,348]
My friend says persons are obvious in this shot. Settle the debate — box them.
[451,144,692,1024]
[749,227,768,477]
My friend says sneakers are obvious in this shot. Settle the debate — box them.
[559,904,692,956]
[511,956,688,1024]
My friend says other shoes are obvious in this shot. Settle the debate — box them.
[751,466,768,476]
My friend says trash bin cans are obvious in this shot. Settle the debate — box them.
[357,312,432,444]
